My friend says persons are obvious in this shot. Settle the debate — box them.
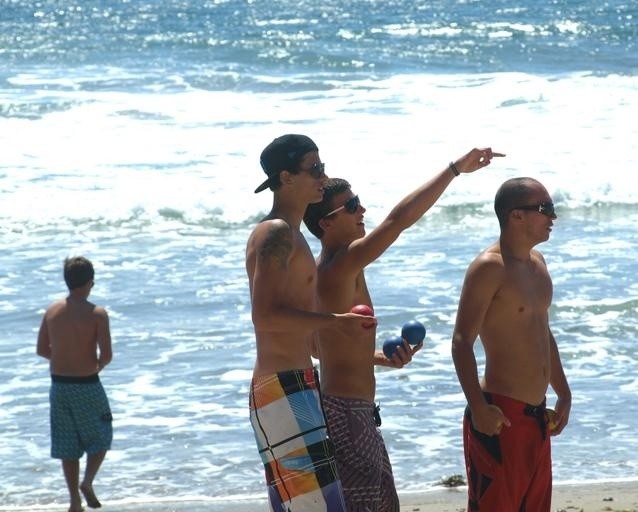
[452,177,573,512]
[308,145,506,511]
[244,134,376,512]
[37,254,119,511]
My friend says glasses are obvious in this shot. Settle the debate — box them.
[322,194,361,218]
[506,203,555,218]
[294,162,325,179]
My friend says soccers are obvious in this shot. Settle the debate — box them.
[383,336,407,361]
[401,320,425,345]
[488,405,503,428]
[545,409,561,431]
[349,305,373,328]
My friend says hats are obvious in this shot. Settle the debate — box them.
[254,132,320,193]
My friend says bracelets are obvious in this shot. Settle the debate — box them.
[448,162,462,178]
